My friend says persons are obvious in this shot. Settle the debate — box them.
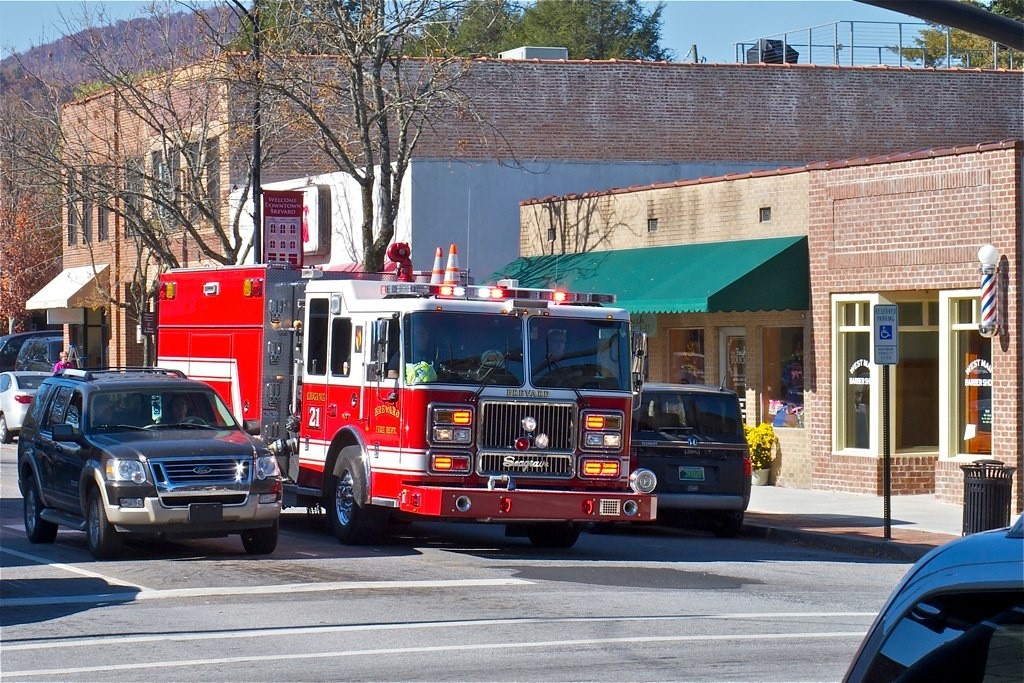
[53,351,73,376]
[169,395,201,425]
[534,329,585,377]
[318,326,350,375]
[94,403,114,425]
[387,323,432,379]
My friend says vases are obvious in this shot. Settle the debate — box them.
[751,468,770,485]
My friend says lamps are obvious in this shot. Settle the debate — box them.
[978,243,1004,338]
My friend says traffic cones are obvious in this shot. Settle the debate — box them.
[443,243,461,285]
[430,246,446,285]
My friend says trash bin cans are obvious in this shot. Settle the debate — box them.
[960,459,1017,537]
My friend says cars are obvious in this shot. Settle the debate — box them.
[0,370,57,443]
[841,513,1024,683]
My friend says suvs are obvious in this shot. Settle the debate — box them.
[18,367,283,559]
[0,329,63,373]
[585,382,752,537]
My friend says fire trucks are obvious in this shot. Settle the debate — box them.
[157,263,658,551]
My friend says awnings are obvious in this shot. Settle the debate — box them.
[474,236,809,313]
[25,264,110,309]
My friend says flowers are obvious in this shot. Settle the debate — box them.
[743,422,780,473]
